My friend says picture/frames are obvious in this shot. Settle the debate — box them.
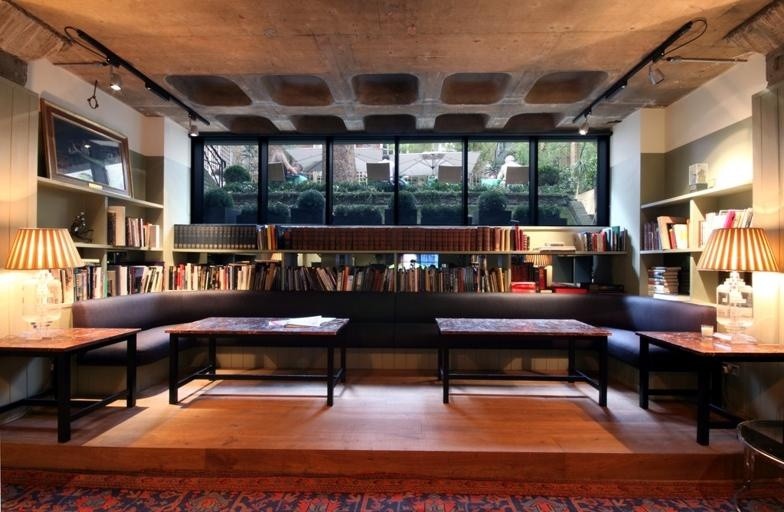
[40,97,136,199]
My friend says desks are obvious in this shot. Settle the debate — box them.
[0,327,142,443]
[637,329,784,447]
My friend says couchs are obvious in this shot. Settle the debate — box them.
[397,291,724,396]
[72,291,397,396]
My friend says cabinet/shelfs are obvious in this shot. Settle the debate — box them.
[168,221,626,293]
[33,176,164,297]
[638,184,756,319]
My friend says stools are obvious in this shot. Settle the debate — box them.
[730,421,784,512]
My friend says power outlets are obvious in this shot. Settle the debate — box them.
[49,363,58,371]
[723,362,731,374]
[732,365,739,376]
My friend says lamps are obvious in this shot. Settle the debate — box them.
[190,125,198,137]
[579,123,593,137]
[110,73,124,91]
[695,227,784,344]
[649,67,665,85]
[5,227,86,343]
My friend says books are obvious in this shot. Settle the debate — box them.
[172,223,531,251]
[700,209,753,246]
[643,216,689,250]
[169,260,281,291]
[646,265,690,295]
[50,258,103,303]
[109,205,159,250]
[396,256,508,293]
[284,254,394,293]
[105,261,164,297]
[511,267,588,293]
[539,227,627,252]
[283,315,336,328]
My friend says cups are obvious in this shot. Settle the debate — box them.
[700,324,715,340]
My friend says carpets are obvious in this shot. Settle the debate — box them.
[1,466,784,512]
